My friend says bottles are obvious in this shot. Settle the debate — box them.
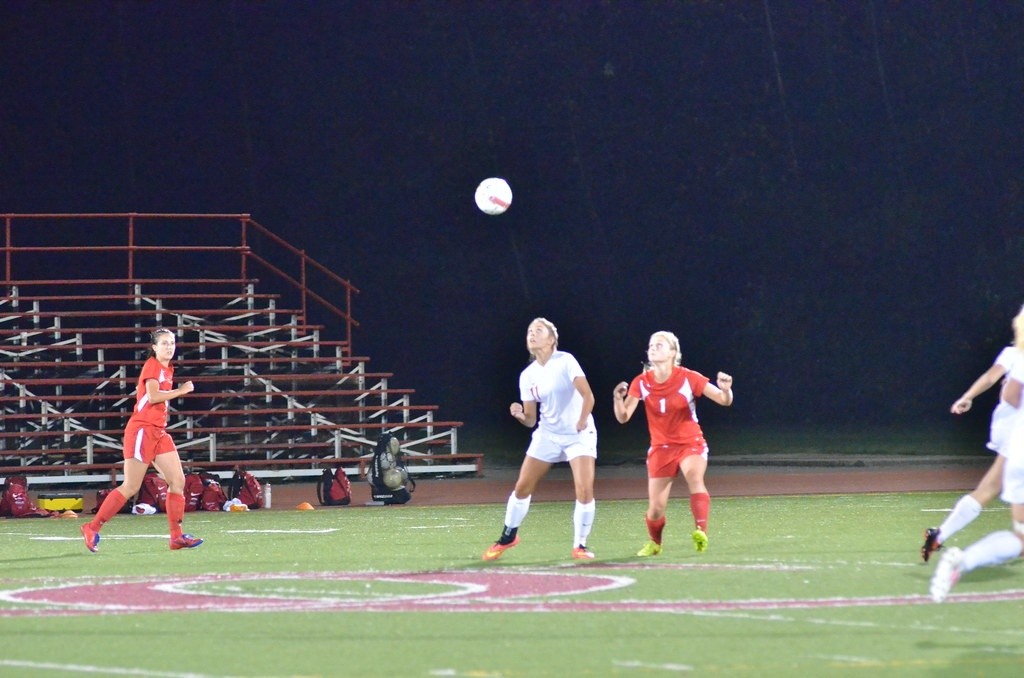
[264,484,271,509]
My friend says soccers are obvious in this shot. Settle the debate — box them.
[380,437,409,489]
[474,177,515,216]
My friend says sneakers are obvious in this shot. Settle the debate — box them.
[483,534,520,562]
[691,530,708,552]
[170,533,204,550]
[930,546,966,603]
[637,539,663,557]
[573,544,594,559]
[922,528,944,562]
[80,523,100,552]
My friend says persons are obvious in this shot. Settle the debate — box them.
[923,307,1023,601]
[483,317,598,561]
[80,328,203,553]
[614,330,733,558]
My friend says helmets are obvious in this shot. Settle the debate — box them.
[382,469,402,488]
[386,437,400,456]
[380,451,397,469]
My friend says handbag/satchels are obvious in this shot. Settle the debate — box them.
[0,475,49,518]
[91,487,134,515]
[131,503,156,516]
[227,464,263,510]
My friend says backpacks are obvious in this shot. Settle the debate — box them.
[136,472,227,511]
[317,467,352,505]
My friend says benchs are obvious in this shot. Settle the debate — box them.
[0,277,484,484]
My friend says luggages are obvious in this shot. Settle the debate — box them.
[37,492,83,513]
